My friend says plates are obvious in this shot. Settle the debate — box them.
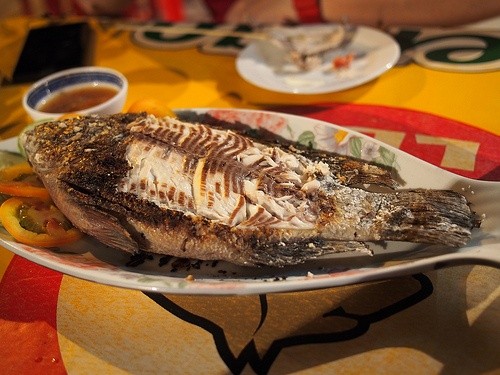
[0,106,500,296]
[235,24,401,95]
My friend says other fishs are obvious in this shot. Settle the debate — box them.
[23,113,484,268]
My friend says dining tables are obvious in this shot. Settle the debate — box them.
[0,14,500,375]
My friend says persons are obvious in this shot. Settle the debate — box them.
[18,1,500,27]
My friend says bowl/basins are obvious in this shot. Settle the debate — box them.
[21,66,128,121]
